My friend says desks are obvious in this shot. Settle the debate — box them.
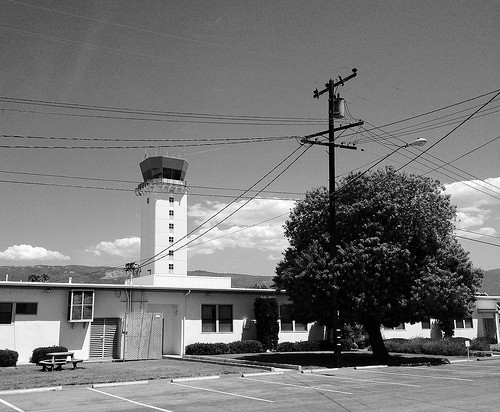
[46,352,76,372]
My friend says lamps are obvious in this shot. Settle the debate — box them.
[206,291,211,296]
[45,288,51,293]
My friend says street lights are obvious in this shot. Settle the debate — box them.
[331,136,427,363]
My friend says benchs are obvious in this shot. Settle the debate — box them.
[72,359,83,363]
[39,358,67,372]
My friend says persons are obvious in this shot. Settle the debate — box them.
[27,273,49,284]
